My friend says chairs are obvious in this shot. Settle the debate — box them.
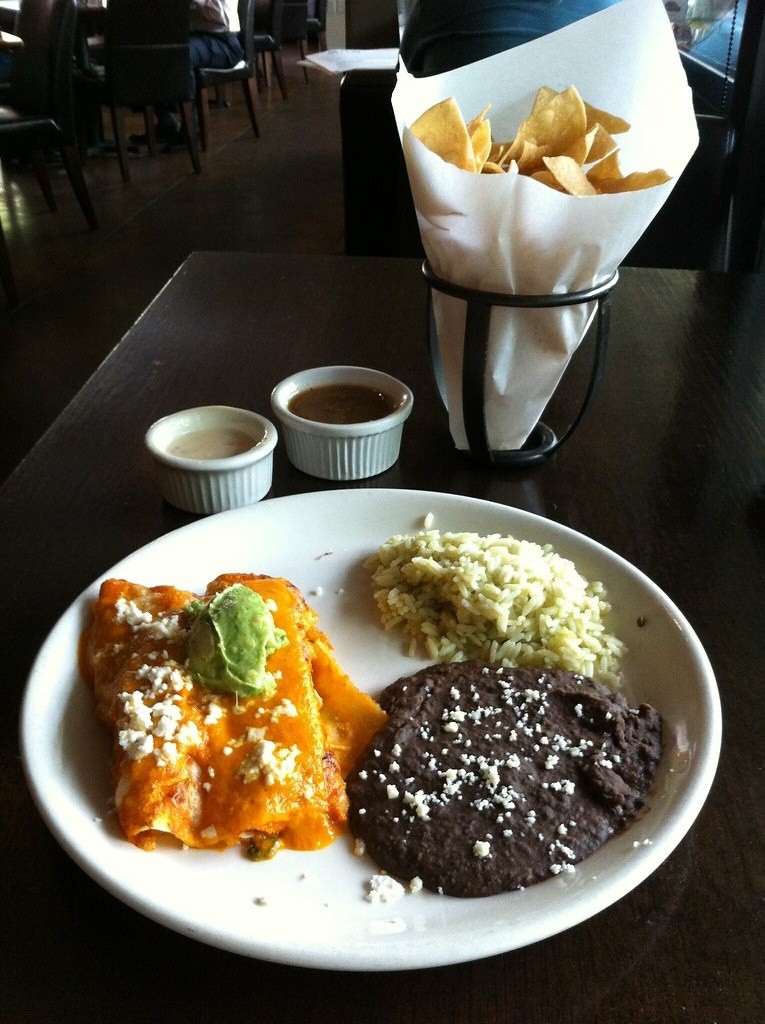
[224,0,328,106]
[340,71,737,271]
[75,0,203,183]
[0,0,98,309]
[195,0,261,151]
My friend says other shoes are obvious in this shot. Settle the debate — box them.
[128,112,180,143]
[166,128,201,153]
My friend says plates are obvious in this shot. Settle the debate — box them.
[20,489,725,968]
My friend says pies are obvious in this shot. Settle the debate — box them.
[90,572,384,855]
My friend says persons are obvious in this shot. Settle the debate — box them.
[385,0,610,81]
[0,0,62,122]
[665,1,692,53]
[130,0,242,146]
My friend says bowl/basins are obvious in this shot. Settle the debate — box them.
[145,405,278,516]
[271,365,414,480]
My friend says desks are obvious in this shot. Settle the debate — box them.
[0,248,765,1023]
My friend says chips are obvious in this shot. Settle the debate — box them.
[407,85,676,195]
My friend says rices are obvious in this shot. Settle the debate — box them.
[360,512,629,694]
